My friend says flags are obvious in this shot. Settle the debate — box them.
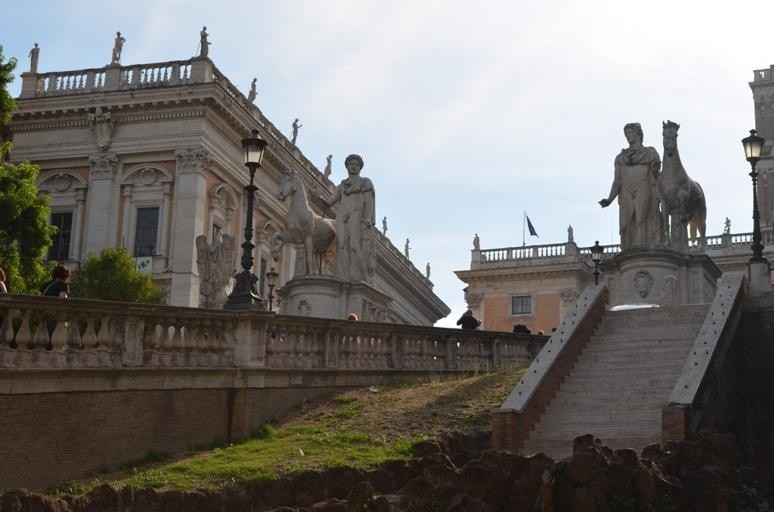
[525,214,539,239]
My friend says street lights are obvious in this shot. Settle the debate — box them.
[221,128,270,311]
[739,127,771,283]
[589,239,605,286]
[263,264,279,310]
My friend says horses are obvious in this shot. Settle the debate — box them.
[271,169,336,276]
[656,120,707,252]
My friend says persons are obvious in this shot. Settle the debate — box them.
[248,77,259,104]
[309,153,378,287]
[29,41,40,72]
[598,122,661,250]
[112,31,126,64]
[323,154,334,179]
[381,216,431,279]
[456,309,482,332]
[291,118,304,145]
[198,26,213,58]
[473,233,481,250]
[0,261,75,298]
